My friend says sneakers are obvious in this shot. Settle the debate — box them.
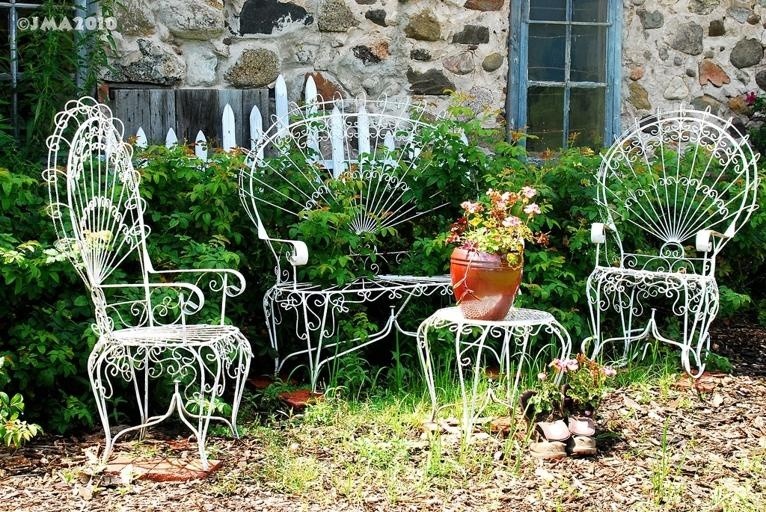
[561,382,597,452]
[518,388,569,460]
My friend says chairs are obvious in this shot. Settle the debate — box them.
[40,90,763,479]
